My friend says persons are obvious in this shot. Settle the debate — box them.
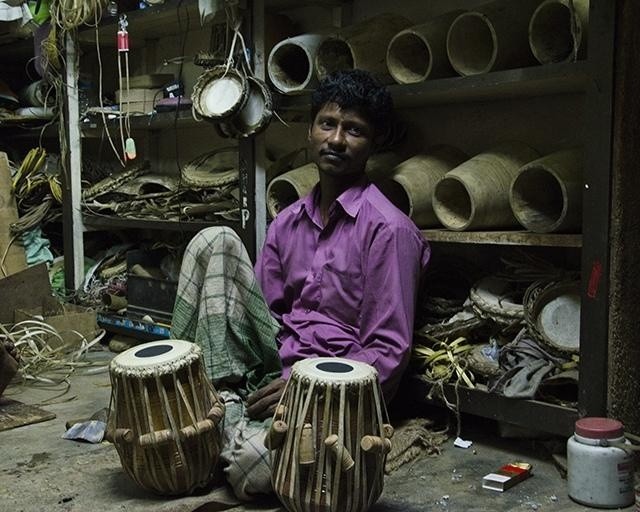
[168,70,432,501]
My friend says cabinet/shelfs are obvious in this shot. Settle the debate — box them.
[0,0,640,438]
